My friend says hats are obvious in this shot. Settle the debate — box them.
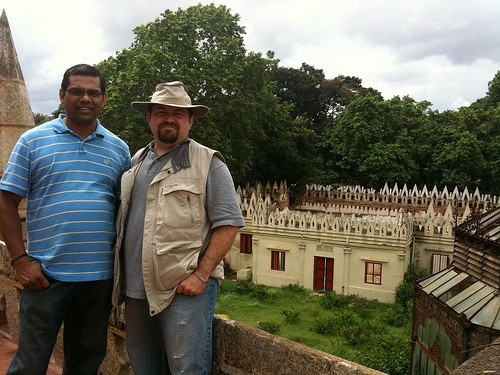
[130,81,210,120]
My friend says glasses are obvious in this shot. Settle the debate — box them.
[64,88,105,98]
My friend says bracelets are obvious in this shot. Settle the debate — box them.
[11,253,28,265]
[194,271,208,284]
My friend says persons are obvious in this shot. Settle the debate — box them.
[111,81,246,375]
[0,64,132,375]
